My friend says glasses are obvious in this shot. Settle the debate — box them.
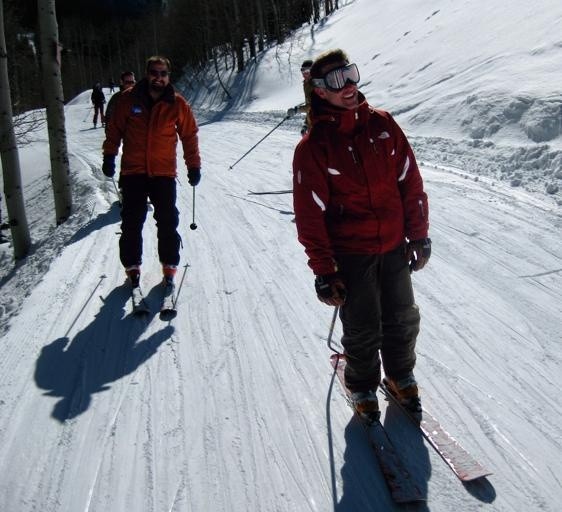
[148,68,169,77]
[122,80,135,85]
[300,67,312,75]
[311,64,359,92]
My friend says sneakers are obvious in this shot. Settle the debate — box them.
[162,265,178,279]
[348,388,379,416]
[383,376,418,401]
[124,265,140,277]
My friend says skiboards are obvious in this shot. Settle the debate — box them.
[329,354,493,504]
[129,278,177,315]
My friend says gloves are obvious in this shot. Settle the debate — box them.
[102,155,116,178]
[405,237,432,272]
[300,126,308,135]
[187,170,201,186]
[287,106,296,117]
[314,274,346,307]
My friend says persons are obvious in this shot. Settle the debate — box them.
[293,49,432,416]
[91,57,201,276]
[288,61,313,136]
